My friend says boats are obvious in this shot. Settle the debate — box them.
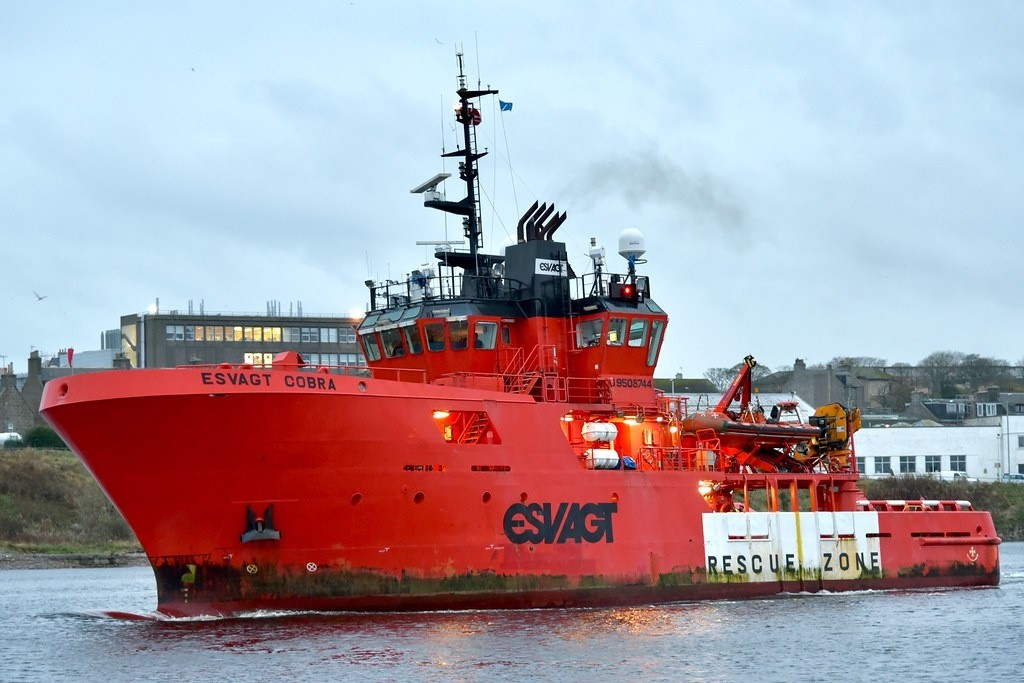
[38,31,1002,618]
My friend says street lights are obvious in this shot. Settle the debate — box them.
[1006,393,1012,483]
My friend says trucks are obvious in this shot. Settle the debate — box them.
[941,471,977,483]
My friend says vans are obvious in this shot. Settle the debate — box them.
[1001,473,1024,484]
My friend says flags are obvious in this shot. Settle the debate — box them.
[500,101,512,110]
